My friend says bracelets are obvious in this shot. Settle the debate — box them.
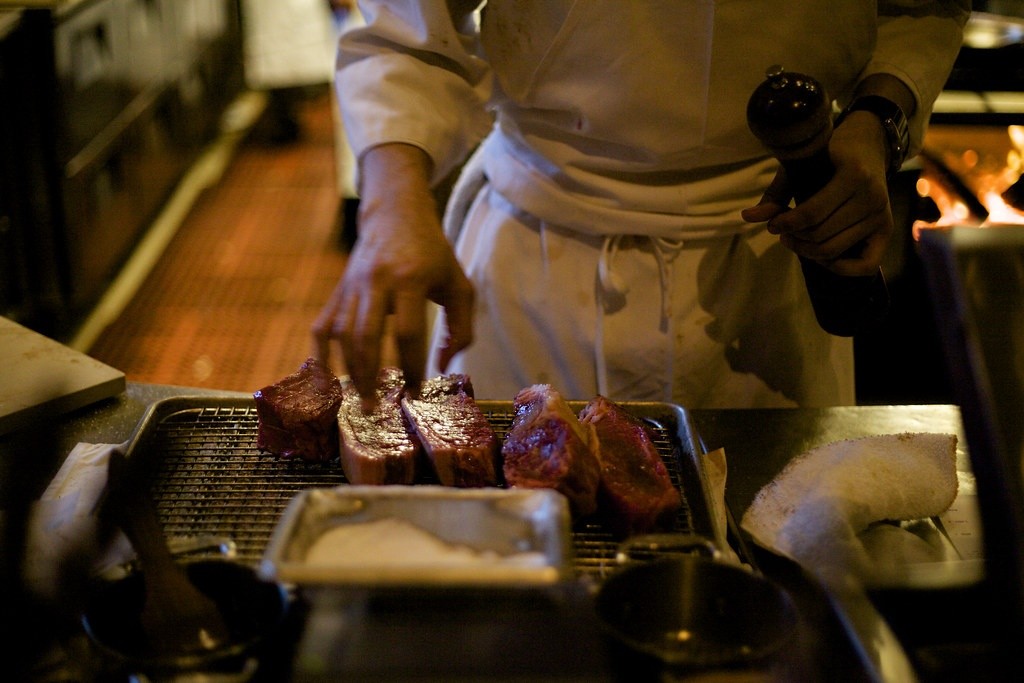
[849,95,910,173]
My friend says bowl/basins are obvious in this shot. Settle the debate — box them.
[81,560,288,671]
[594,556,800,667]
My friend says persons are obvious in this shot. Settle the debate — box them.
[307,0,972,416]
